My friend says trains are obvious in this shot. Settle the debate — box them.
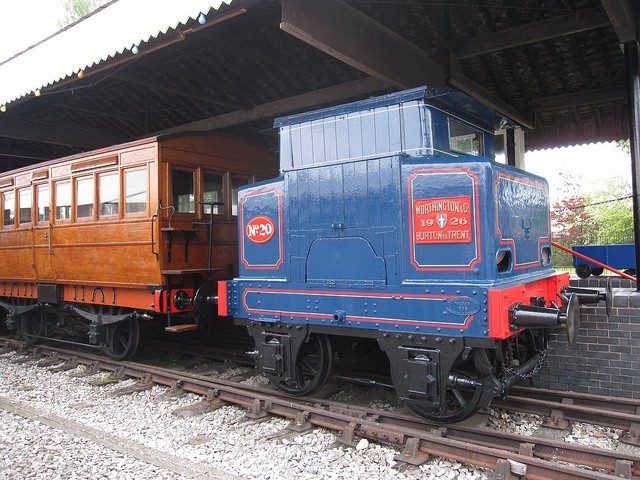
[572,242,636,279]
[0,85,612,424]
